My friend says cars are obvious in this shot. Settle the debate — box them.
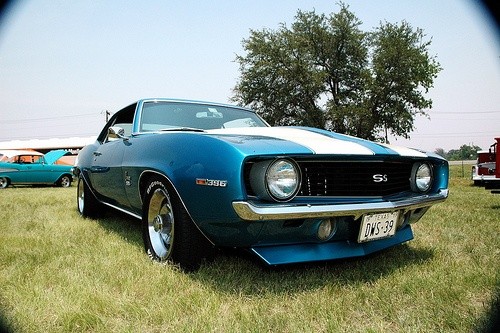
[0,148,74,189]
[75,98,450,275]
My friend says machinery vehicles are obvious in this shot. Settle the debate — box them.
[471,137,500,196]
